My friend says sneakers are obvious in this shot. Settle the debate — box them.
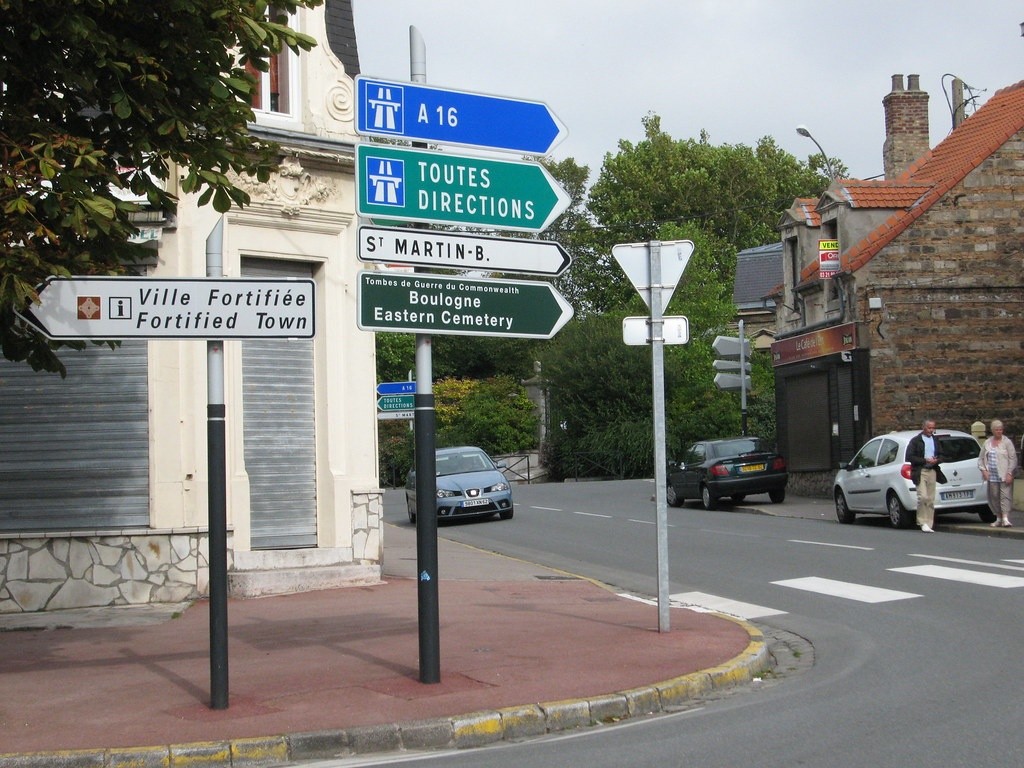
[990,521,1000,527]
[1000,521,1012,527]
[921,524,934,533]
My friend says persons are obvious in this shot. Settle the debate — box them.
[905,418,948,532]
[977,419,1018,527]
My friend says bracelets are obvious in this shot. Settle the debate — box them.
[1008,471,1012,477]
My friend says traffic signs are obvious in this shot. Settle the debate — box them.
[377,380,415,423]
[350,72,578,341]
[14,267,318,344]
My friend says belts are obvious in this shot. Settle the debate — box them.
[922,466,935,469]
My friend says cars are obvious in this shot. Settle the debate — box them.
[404,444,515,525]
[833,430,997,531]
[667,435,790,511]
